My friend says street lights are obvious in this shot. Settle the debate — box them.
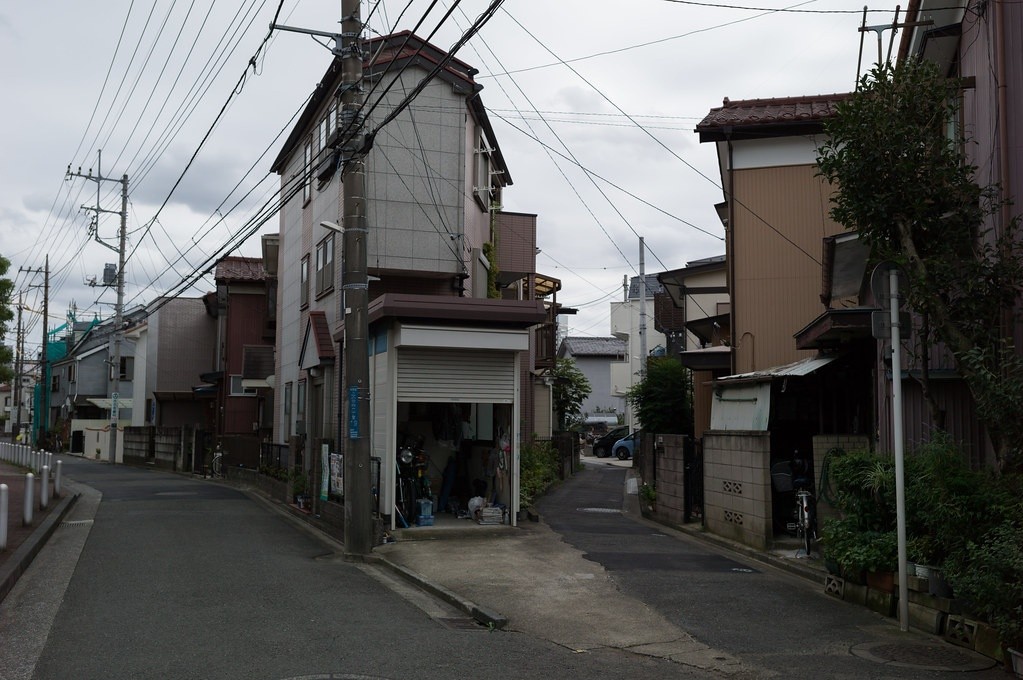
[321,219,347,503]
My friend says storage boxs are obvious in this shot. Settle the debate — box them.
[416,516,434,525]
[416,499,433,515]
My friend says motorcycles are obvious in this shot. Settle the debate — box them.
[397,436,436,521]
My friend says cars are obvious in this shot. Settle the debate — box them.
[594,427,638,459]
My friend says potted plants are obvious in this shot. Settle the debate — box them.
[822,518,985,620]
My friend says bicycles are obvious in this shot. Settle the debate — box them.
[774,446,817,557]
[210,442,226,476]
